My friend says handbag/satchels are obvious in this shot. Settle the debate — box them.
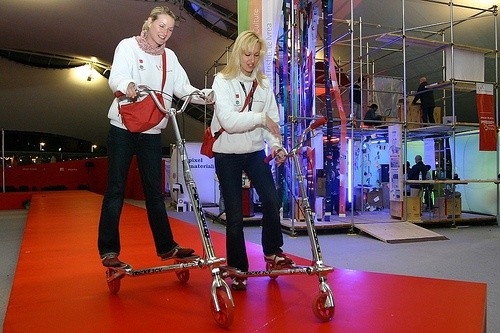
[200,127,215,159]
[119,93,166,133]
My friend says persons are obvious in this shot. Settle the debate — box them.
[98,6,216,267]
[364,104,386,129]
[403,161,411,196]
[408,155,427,216]
[424,164,434,213]
[211,30,293,291]
[349,77,365,128]
[412,77,435,127]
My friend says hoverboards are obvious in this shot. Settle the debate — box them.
[106,85,235,328]
[219,116,335,323]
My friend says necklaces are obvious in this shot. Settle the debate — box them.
[240,81,254,111]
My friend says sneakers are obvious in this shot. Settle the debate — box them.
[160,246,195,260]
[264,252,295,267]
[231,277,248,291]
[102,255,133,272]
